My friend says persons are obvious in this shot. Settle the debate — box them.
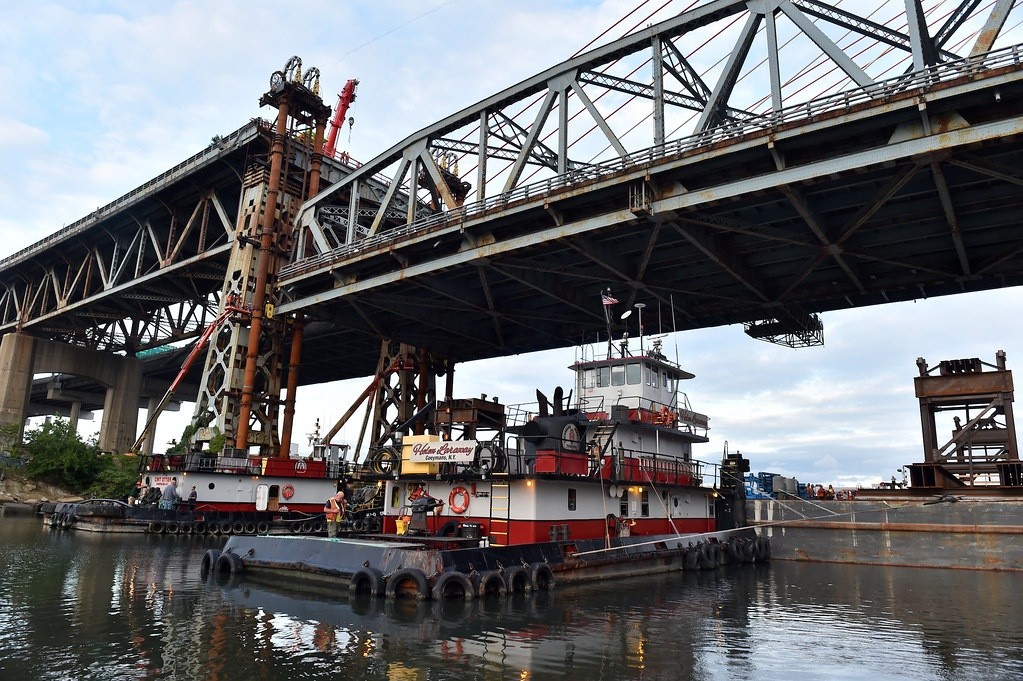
[187,485,196,516]
[127,481,146,506]
[323,491,347,538]
[805,483,848,501]
[161,480,179,509]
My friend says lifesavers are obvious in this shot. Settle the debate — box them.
[662,406,670,426]
[282,484,295,499]
[449,487,469,514]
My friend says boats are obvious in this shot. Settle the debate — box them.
[41,417,385,548]
[202,287,771,599]
